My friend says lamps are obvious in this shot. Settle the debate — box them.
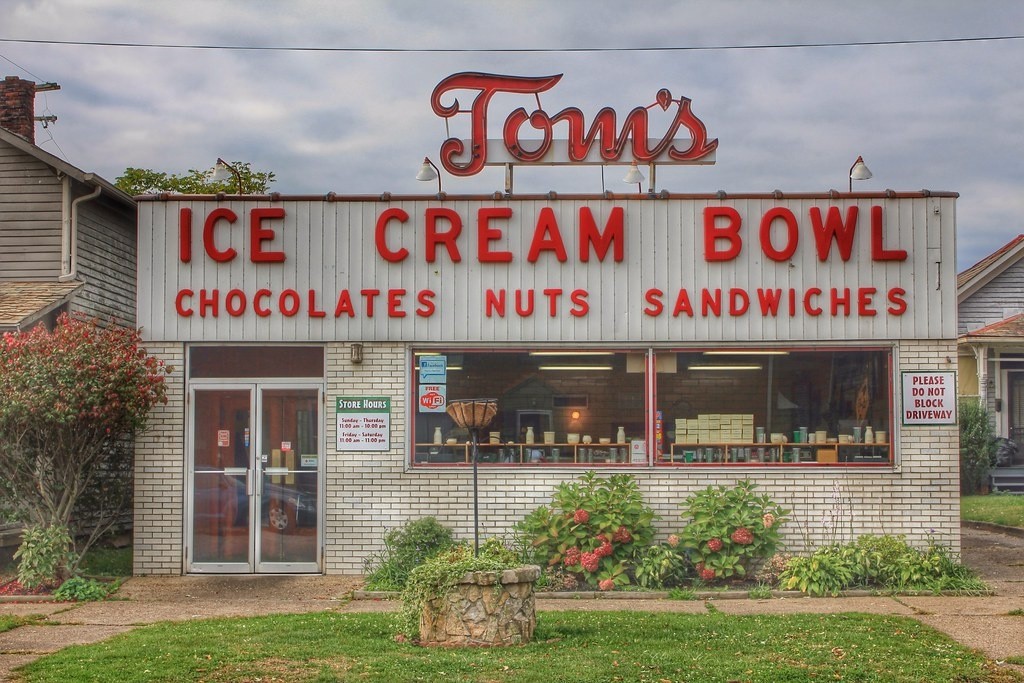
[849,155,873,193]
[414,156,448,200]
[622,159,645,193]
[210,157,242,195]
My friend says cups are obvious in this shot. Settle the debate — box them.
[684,448,800,462]
[524,448,532,462]
[770,432,783,443]
[552,449,560,463]
[838,435,848,444]
[489,431,501,444]
[816,430,827,443]
[610,448,617,462]
[875,431,886,444]
[566,433,580,444]
[793,431,801,443]
[544,431,556,444]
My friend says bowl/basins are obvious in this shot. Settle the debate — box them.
[445,437,457,444]
[600,437,611,444]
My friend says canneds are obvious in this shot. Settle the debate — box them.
[799,427,808,442]
[756,427,764,442]
[793,448,800,462]
[509,449,516,463]
[619,448,627,463]
[497,449,505,463]
[716,448,723,462]
[696,448,704,462]
[587,449,593,462]
[610,448,617,463]
[770,447,776,461]
[552,449,560,462]
[706,448,713,462]
[730,448,738,462]
[524,449,532,462]
[744,448,751,462]
[853,427,861,443]
[757,448,765,462]
[579,449,586,463]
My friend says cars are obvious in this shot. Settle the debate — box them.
[194,466,317,534]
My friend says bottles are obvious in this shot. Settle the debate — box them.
[433,426,442,444]
[497,448,515,463]
[617,427,626,444]
[865,426,873,443]
[526,426,535,444]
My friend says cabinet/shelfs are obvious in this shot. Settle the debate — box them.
[781,443,837,463]
[415,444,468,462]
[520,444,577,464]
[466,443,522,464]
[574,444,631,463]
[836,442,890,462]
[670,443,727,463]
[725,443,781,462]
